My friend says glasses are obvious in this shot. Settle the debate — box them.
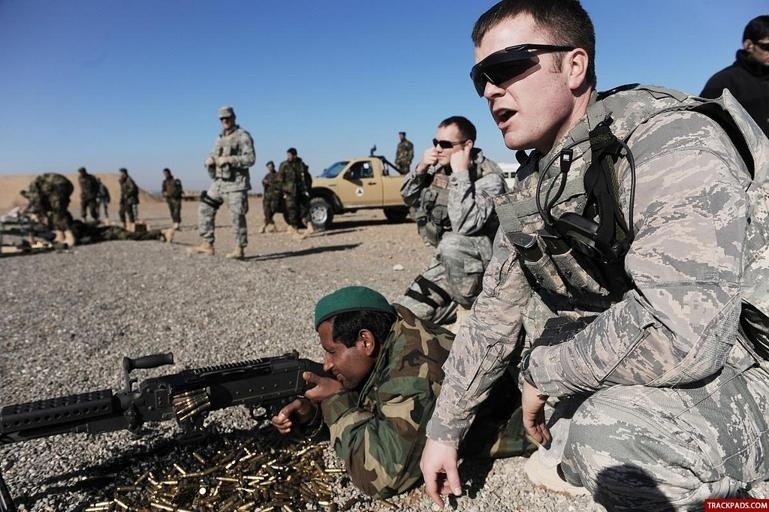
[433,138,466,148]
[754,42,768,52]
[470,44,576,97]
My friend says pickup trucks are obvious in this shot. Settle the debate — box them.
[274,157,417,229]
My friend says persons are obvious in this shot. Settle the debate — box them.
[271,285,549,501]
[119,168,140,226]
[23,173,74,247]
[259,147,314,238]
[696,12,769,140]
[162,168,183,229]
[196,107,256,257]
[395,132,414,172]
[70,220,175,242]
[419,1,769,512]
[78,168,110,225]
[400,116,510,325]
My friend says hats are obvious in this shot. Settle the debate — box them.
[219,107,234,118]
[314,285,397,329]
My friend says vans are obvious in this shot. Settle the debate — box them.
[494,163,523,190]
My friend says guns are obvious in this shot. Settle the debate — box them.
[0,352,337,510]
[0,219,56,241]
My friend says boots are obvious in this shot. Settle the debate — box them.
[195,239,214,254]
[301,222,315,238]
[227,245,244,258]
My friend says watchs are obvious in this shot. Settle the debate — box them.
[520,349,536,389]
